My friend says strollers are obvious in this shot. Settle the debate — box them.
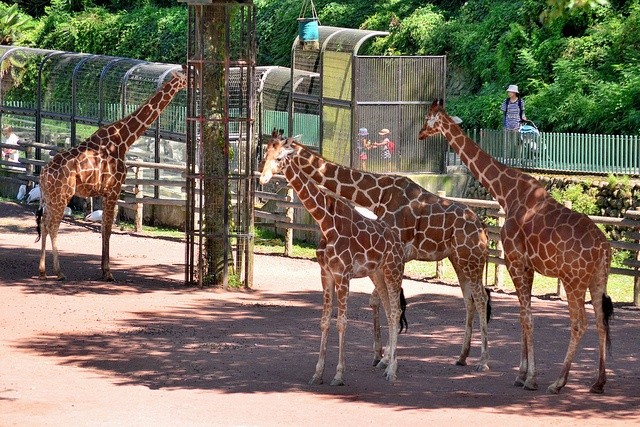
[517,119,553,167]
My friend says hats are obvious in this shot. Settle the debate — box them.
[356,127,369,136]
[379,129,390,135]
[505,85,519,93]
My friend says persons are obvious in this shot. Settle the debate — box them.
[352,128,371,172]
[2,125,19,165]
[372,129,395,173]
[500,84,527,168]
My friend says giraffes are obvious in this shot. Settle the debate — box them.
[258,128,409,387]
[418,99,614,395]
[34,64,199,282]
[259,127,492,373]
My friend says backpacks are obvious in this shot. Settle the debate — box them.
[351,138,365,155]
[386,141,396,155]
